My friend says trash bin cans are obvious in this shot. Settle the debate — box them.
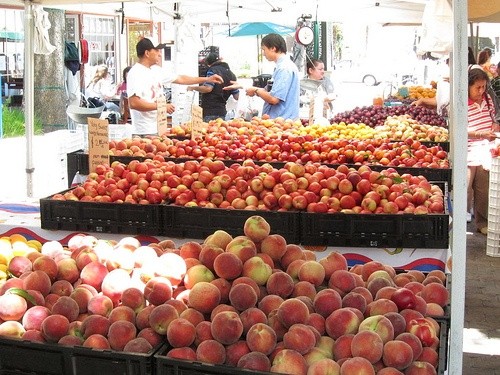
[66,148,89,189]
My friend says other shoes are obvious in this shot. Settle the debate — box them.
[482,226,487,235]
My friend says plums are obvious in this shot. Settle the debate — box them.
[329,105,448,127]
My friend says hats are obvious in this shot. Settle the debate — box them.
[202,52,219,66]
[136,37,166,50]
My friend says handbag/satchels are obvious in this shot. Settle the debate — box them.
[418,0,454,53]
[224,91,265,122]
[33,5,57,56]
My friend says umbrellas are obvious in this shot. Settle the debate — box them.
[218,22,296,75]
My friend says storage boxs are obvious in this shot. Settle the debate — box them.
[0,141,452,375]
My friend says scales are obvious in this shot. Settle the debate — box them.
[293,13,336,102]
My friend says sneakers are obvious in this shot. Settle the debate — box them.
[467,208,474,223]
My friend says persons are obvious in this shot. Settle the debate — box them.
[299,60,338,118]
[86,65,132,124]
[407,47,500,235]
[187,45,239,123]
[126,37,224,140]
[223,33,300,118]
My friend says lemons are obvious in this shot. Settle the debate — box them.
[292,121,374,140]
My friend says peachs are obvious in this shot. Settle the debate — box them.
[53,154,444,215]
[0,216,449,375]
[108,135,450,168]
[166,114,303,135]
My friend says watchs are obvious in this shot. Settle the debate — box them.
[254,89,259,96]
